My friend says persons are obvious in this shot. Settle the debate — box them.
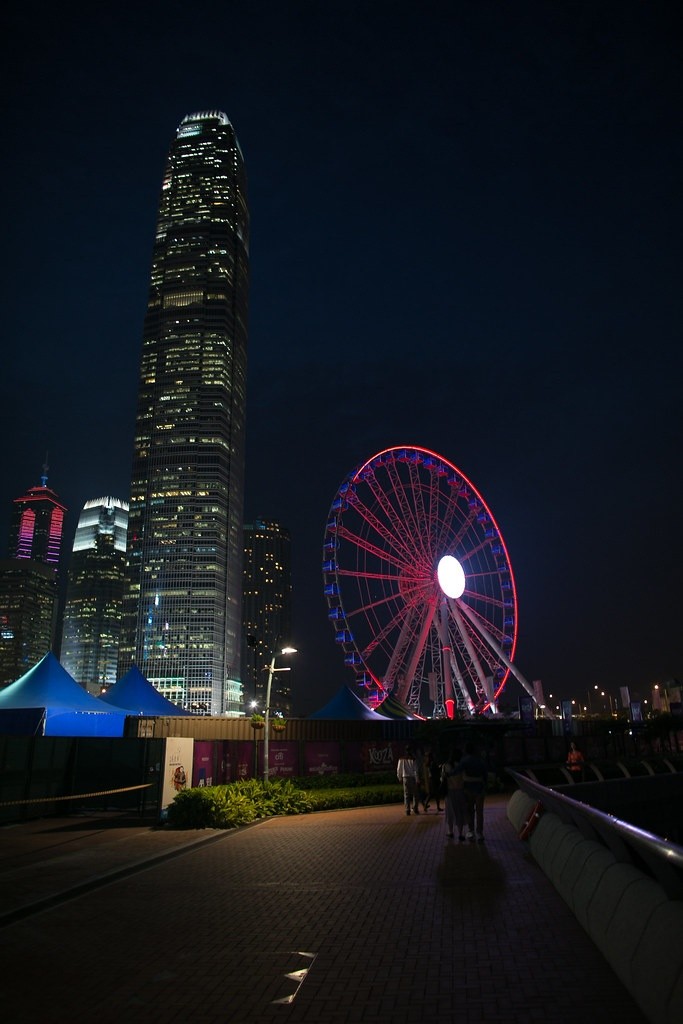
[396,745,488,843]
[567,741,586,783]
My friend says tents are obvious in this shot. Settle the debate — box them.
[0,650,198,737]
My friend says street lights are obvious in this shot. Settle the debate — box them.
[262,646,298,781]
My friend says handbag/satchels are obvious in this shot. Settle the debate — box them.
[437,761,447,798]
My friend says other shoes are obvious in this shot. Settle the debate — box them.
[411,807,419,814]
[477,835,485,844]
[424,805,430,808]
[458,835,465,841]
[465,831,476,841]
[436,807,443,811]
[405,810,410,816]
[444,832,453,839]
[423,808,428,812]
